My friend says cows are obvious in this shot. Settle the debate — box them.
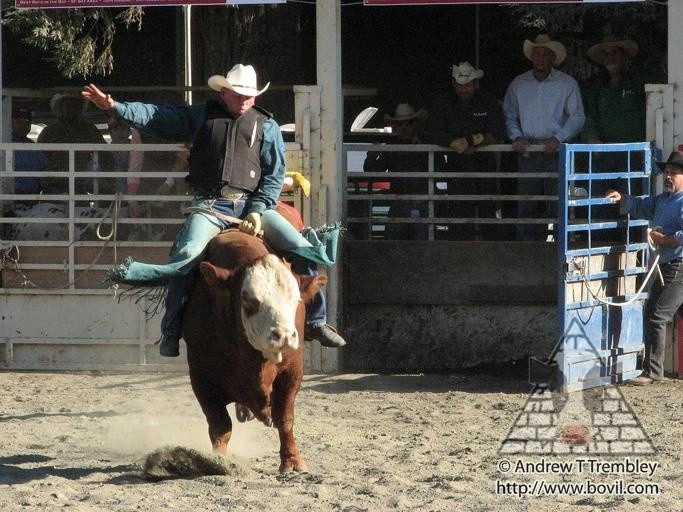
[180,228,328,475]
[2,203,168,241]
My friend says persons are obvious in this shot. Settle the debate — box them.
[430,62,504,241]
[78,62,348,359]
[362,103,428,241]
[579,34,647,219]
[604,151,683,387]
[501,34,586,241]
[12,31,214,241]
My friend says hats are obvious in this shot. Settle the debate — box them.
[11,106,32,122]
[586,33,641,65]
[383,103,429,128]
[207,63,271,97]
[522,33,567,66]
[449,60,484,85]
[49,89,88,116]
[107,117,130,131]
[655,151,683,172]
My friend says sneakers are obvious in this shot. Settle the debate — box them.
[304,321,348,348]
[630,371,664,386]
[158,331,180,357]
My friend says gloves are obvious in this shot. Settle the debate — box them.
[239,212,260,237]
